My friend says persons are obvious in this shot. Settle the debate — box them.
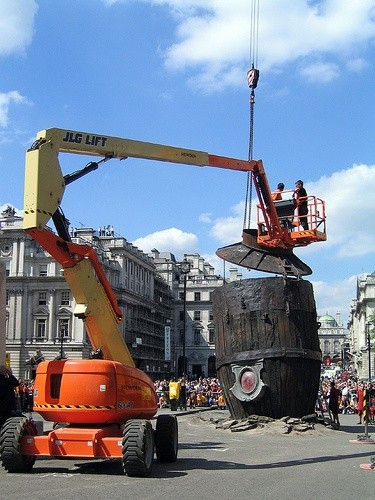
[292,180,309,230]
[272,183,297,229]
[98,225,115,237]
[323,382,342,429]
[316,374,344,414]
[0,364,20,427]
[342,379,375,426]
[153,376,227,410]
[14,378,35,413]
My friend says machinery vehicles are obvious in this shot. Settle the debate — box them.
[0,126,327,478]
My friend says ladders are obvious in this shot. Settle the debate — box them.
[317,382,332,418]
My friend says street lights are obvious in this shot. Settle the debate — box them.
[180,256,192,386]
[59,322,65,359]
[365,322,373,407]
